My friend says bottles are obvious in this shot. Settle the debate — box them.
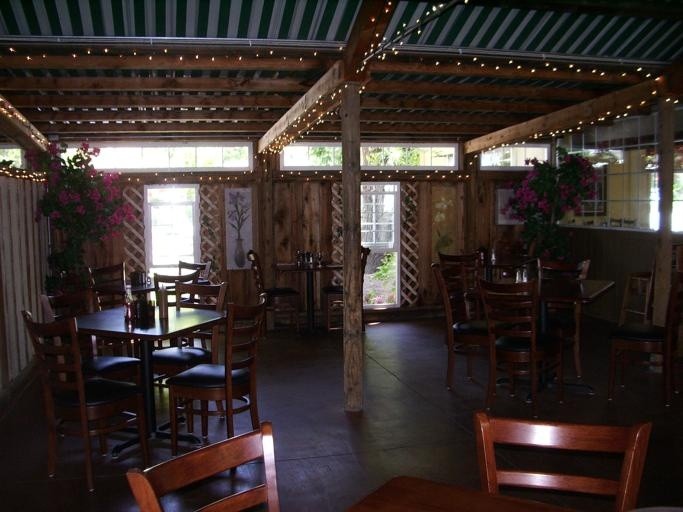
[157,283,167,317]
[514,266,521,283]
[474,247,496,262]
[296,248,321,266]
[522,265,529,282]
[123,285,154,322]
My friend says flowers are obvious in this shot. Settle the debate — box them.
[16,131,137,242]
[225,191,252,240]
[495,141,603,233]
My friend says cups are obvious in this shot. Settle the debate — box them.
[129,271,145,285]
